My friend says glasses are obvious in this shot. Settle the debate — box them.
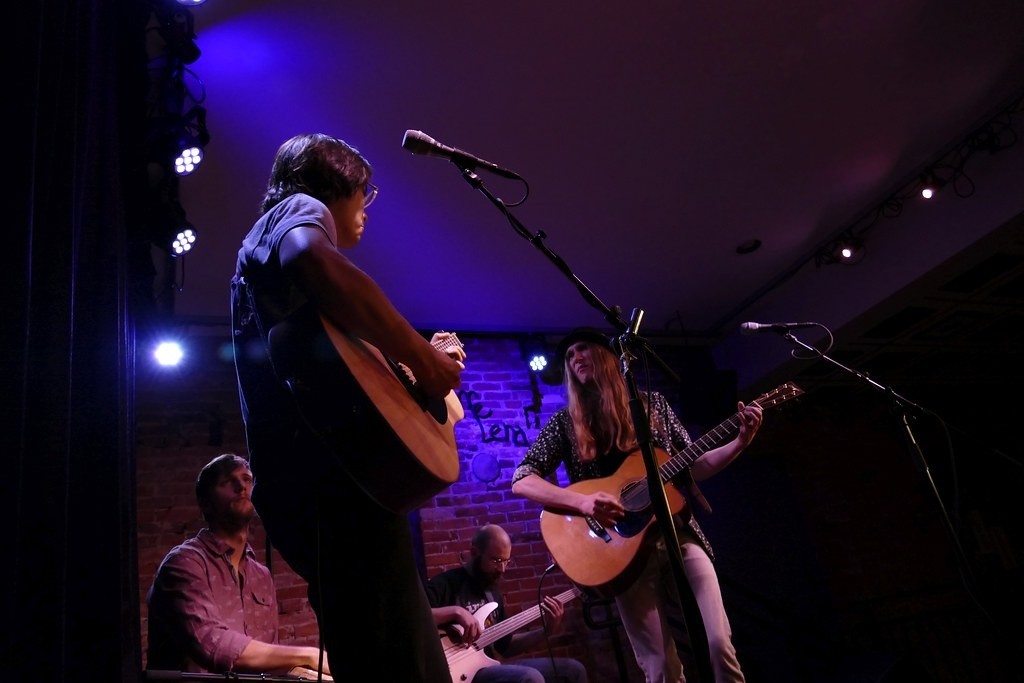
[478,546,512,567]
[363,178,379,210]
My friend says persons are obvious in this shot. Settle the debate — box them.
[229,134,467,683]
[426,524,587,683]
[146,454,333,681]
[513,327,763,683]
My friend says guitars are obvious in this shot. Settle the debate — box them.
[265,302,465,517]
[436,587,582,683]
[536,379,806,601]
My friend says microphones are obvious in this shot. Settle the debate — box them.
[402,129,522,180]
[741,322,818,336]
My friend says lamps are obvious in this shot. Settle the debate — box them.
[139,205,198,257]
[147,121,206,178]
[518,331,549,371]
[920,177,949,198]
[841,237,865,256]
[136,1,201,98]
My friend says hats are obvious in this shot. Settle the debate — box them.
[555,326,620,370]
